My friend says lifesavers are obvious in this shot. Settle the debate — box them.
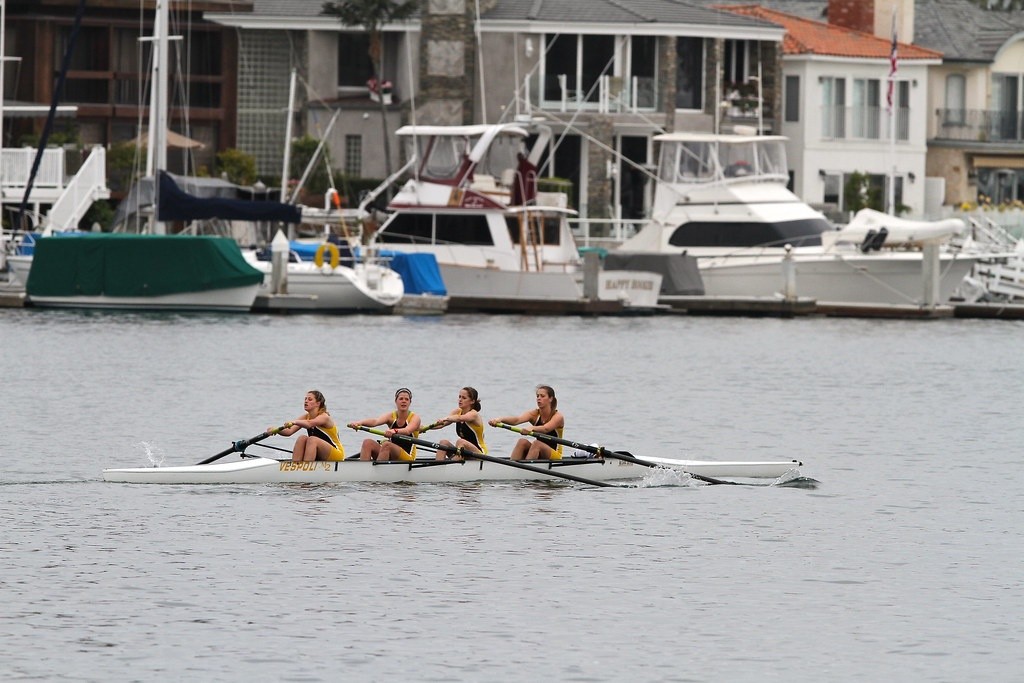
[313,242,340,273]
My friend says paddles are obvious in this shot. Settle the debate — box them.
[194,422,295,464]
[488,421,737,486]
[343,422,446,461]
[347,421,627,490]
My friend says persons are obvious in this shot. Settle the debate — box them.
[487,386,564,460]
[267,391,345,461]
[421,387,488,460]
[352,388,421,460]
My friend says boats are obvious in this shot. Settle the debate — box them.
[596,128,983,310]
[99,454,807,486]
[357,122,665,317]
[4,1,408,314]
[29,233,267,316]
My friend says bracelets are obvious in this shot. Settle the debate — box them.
[394,428,398,434]
